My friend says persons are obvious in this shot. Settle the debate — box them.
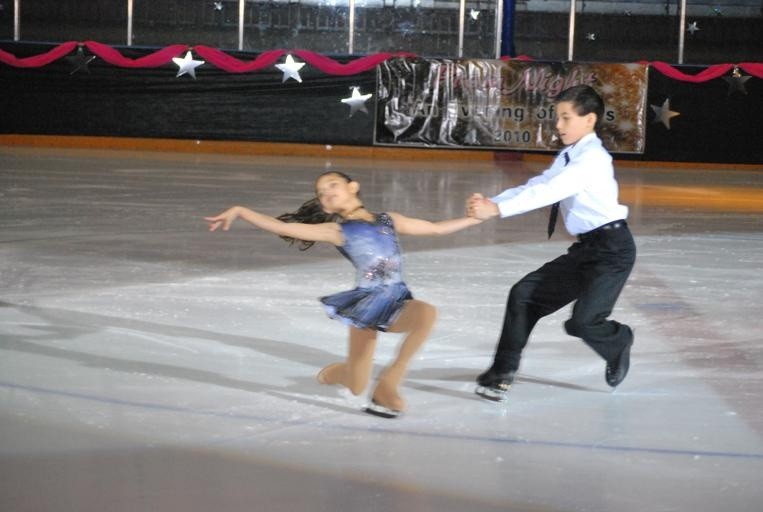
[461,84,640,389]
[200,169,486,413]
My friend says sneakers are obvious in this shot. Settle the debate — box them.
[477,365,514,391]
[371,383,403,411]
[316,362,344,384]
[606,324,632,386]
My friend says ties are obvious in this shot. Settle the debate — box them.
[548,150,569,238]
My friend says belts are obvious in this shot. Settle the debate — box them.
[578,219,625,241]
